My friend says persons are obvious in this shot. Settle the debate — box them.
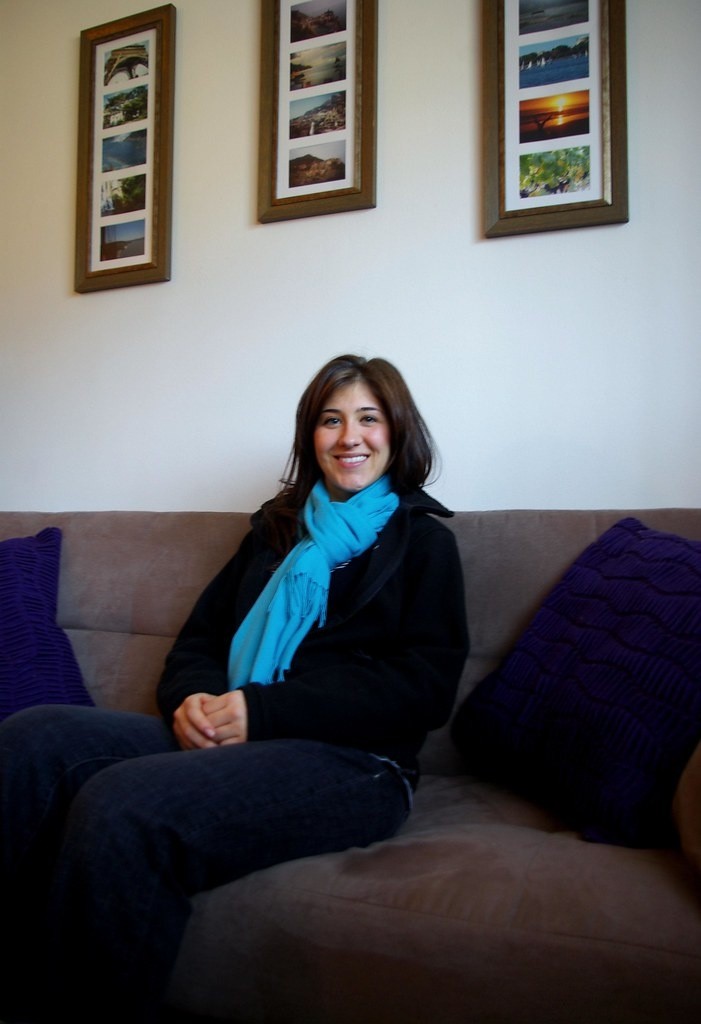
[0,354,470,1024]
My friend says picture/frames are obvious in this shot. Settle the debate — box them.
[73,3,178,295]
[480,0,629,238]
[256,0,379,225]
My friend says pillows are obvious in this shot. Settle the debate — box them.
[449,517,701,850]
[0,526,97,719]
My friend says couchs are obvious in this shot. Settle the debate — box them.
[0,505,701,1024]
